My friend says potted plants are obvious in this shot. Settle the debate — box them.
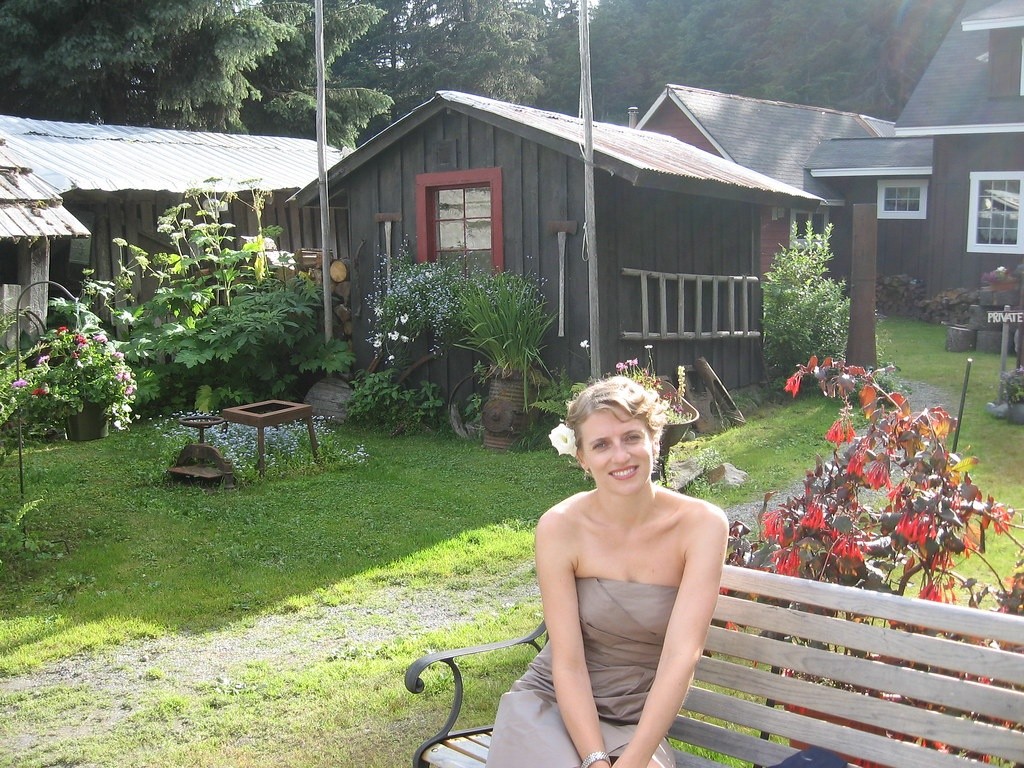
[454,283,559,451]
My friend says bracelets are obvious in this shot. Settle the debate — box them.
[581,752,612,768]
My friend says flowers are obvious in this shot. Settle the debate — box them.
[983,266,1016,282]
[999,365,1024,408]
[580,339,693,422]
[14,326,141,433]
[548,423,577,457]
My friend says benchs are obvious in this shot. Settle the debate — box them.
[406,565,1024,768]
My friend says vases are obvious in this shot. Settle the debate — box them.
[657,381,699,485]
[1010,404,1024,424]
[65,401,108,440]
[990,282,1014,291]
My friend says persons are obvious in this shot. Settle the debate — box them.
[488,378,730,768]
